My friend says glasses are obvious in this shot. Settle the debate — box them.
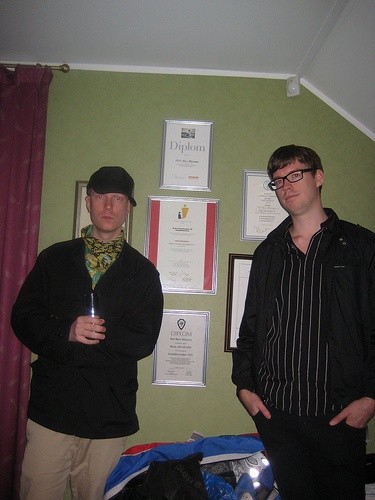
[268,166,315,192]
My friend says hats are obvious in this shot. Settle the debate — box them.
[86,166,137,208]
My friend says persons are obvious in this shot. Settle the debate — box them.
[231,144,375,500]
[9,166,165,500]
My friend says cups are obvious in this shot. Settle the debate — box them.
[84,292,103,322]
